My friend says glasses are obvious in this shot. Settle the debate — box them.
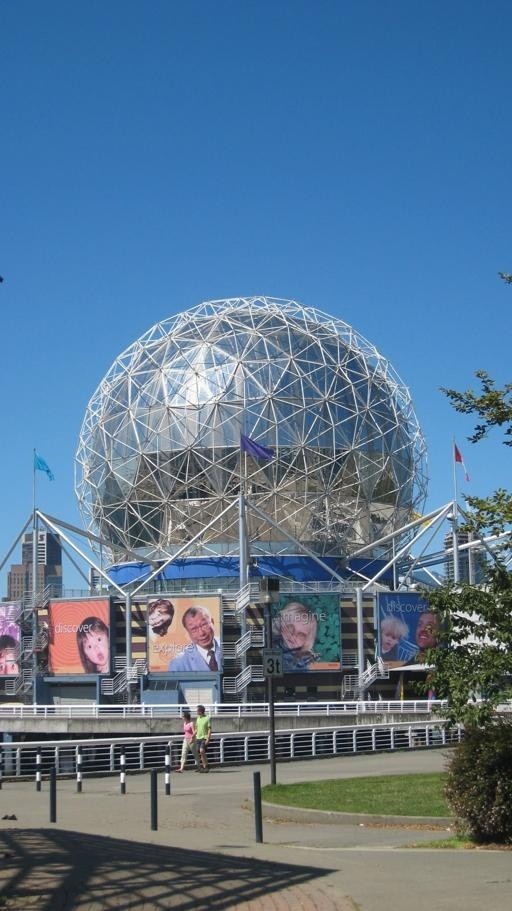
[189,622,210,633]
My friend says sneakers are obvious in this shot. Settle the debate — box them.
[175,769,183,772]
[196,765,210,773]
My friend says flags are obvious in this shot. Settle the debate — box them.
[34,452,54,481]
[239,432,274,461]
[413,511,434,529]
[454,443,470,482]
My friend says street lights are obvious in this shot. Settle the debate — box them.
[257,578,281,781]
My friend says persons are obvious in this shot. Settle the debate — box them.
[148,598,174,637]
[76,617,109,674]
[167,607,222,671]
[272,601,317,670]
[384,610,442,668]
[381,615,409,662]
[175,705,211,773]
[0,634,20,674]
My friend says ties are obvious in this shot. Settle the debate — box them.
[207,649,217,670]
